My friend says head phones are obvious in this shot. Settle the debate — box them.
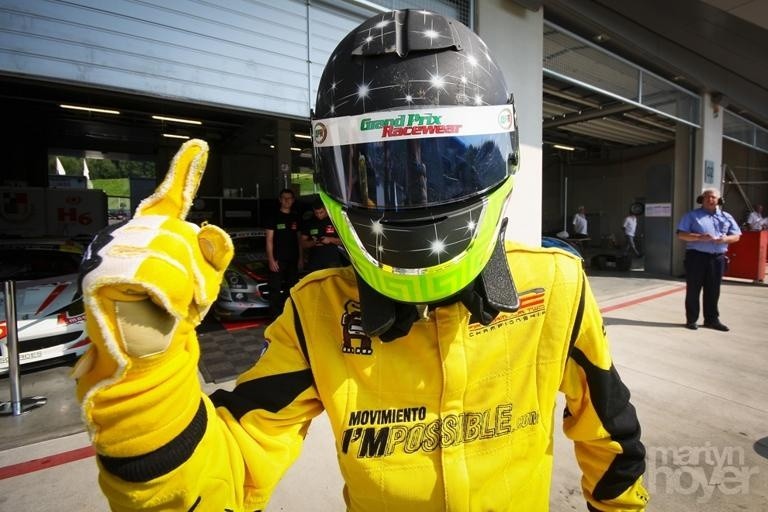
[697,195,726,205]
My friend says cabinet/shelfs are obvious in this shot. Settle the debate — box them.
[644,217,672,279]
[721,228,768,287]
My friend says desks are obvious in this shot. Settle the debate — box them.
[566,237,593,255]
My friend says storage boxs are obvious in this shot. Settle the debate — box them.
[644,202,672,218]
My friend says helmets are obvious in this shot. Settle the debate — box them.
[311,8,519,304]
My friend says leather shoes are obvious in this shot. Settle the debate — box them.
[686,317,729,331]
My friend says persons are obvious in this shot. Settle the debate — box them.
[571,205,590,251]
[344,152,375,208]
[747,203,768,231]
[69,8,653,511]
[676,187,743,332]
[621,206,641,258]
[301,197,345,277]
[265,187,304,322]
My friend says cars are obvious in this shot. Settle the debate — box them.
[1,238,95,377]
[210,207,309,323]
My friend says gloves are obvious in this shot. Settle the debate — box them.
[70,138,235,458]
